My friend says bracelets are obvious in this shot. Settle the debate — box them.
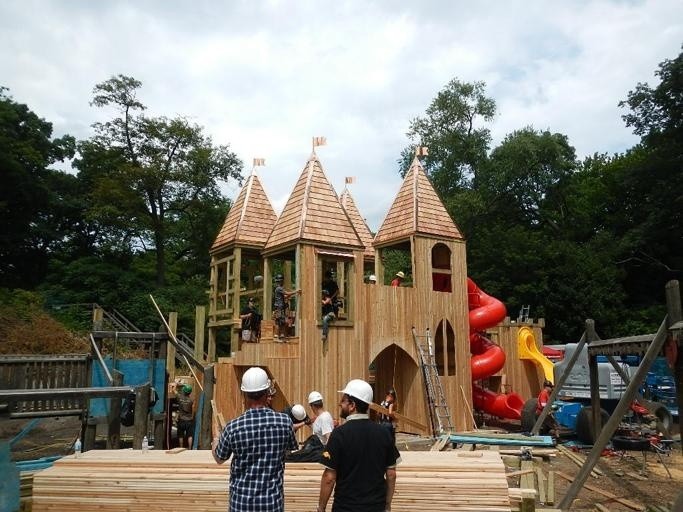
[303,418,311,426]
[175,381,179,383]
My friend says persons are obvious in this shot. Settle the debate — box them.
[390,271,405,287]
[377,389,399,433]
[172,378,194,450]
[265,388,278,413]
[321,290,336,339]
[280,403,315,432]
[307,391,333,447]
[321,270,339,319]
[239,297,260,343]
[535,380,563,443]
[368,275,376,285]
[272,273,302,343]
[211,367,298,512]
[315,378,401,512]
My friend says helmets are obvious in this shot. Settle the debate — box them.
[239,366,271,393]
[274,274,284,282]
[291,404,307,421]
[544,381,555,388]
[182,384,192,395]
[384,388,396,397]
[322,289,330,295]
[336,379,374,406]
[307,391,323,404]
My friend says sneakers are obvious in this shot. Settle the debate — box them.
[321,333,328,340]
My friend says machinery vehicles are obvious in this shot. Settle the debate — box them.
[521,343,678,446]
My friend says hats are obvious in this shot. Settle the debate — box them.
[396,270,405,279]
[368,274,376,282]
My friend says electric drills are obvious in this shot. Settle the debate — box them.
[248,310,263,316]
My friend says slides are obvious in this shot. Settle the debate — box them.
[467,277,525,420]
[530,351,555,386]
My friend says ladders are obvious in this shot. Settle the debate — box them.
[412,327,454,435]
